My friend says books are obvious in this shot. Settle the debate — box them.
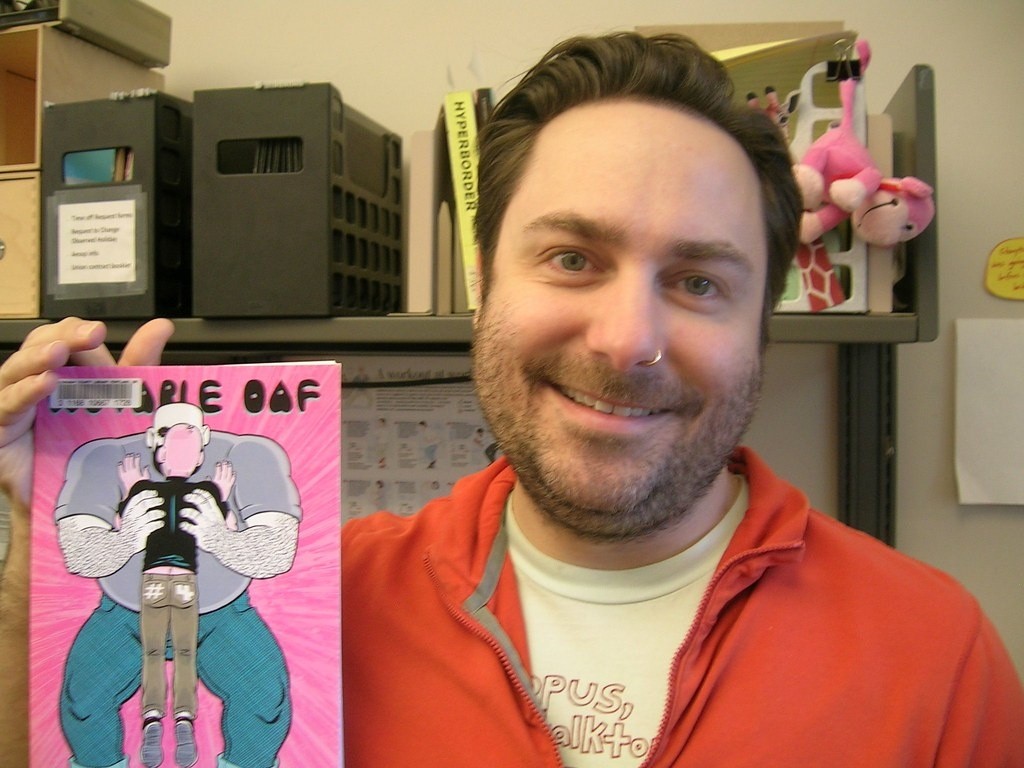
[110,85,156,183]
[429,86,494,315]
[27,360,344,768]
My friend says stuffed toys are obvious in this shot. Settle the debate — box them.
[793,40,933,245]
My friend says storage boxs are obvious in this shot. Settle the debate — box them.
[0,0,173,71]
[188,81,412,320]
[37,91,192,321]
[0,24,166,173]
[0,168,41,323]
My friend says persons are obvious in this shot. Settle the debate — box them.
[0,32,1024,768]
